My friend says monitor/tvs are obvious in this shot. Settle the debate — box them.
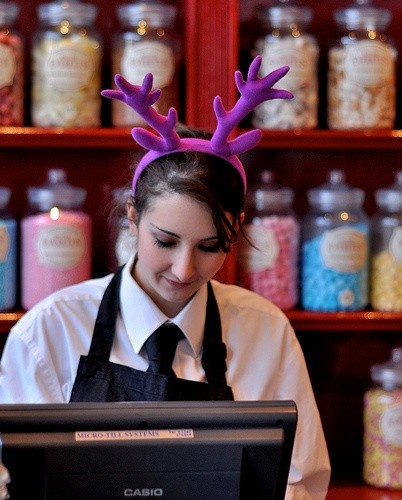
[0,399,298,500]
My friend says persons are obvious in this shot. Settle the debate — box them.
[0,129,331,500]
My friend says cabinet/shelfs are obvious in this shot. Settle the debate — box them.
[0,0,402,331]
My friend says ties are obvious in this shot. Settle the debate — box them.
[144,323,186,380]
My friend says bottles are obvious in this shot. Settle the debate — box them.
[250,1,319,130]
[370,171,402,313]
[0,187,18,312]
[0,3,25,127]
[21,169,93,310]
[111,3,181,127]
[239,171,300,310]
[302,171,371,312]
[106,188,135,273]
[327,1,398,131]
[31,1,103,127]
[363,348,402,489]
